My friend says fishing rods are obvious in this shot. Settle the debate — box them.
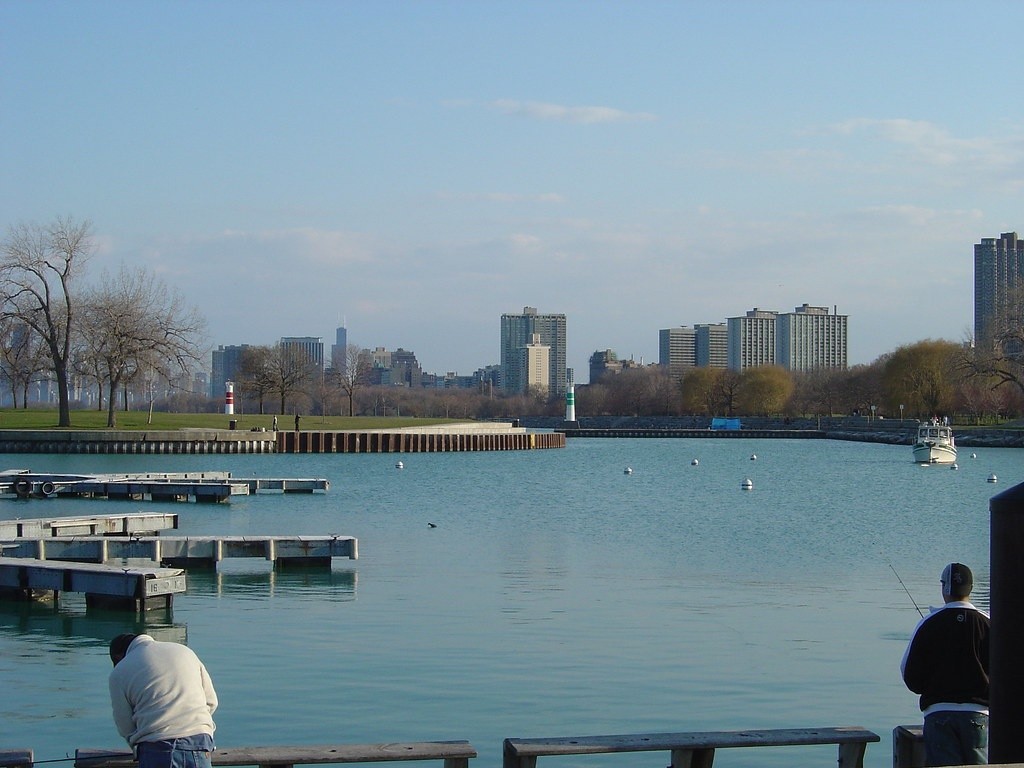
[0,745,217,768]
[889,564,924,618]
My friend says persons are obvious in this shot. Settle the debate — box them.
[109,634,218,768]
[901,563,990,768]
[273,415,279,431]
[295,414,301,431]
[853,408,860,416]
[740,422,746,430]
[933,414,952,426]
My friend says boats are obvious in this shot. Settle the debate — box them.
[912,415,957,463]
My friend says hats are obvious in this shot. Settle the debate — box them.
[942,563,973,596]
[109,633,132,666]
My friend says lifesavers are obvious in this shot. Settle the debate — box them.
[41,481,55,495]
[14,477,32,495]
